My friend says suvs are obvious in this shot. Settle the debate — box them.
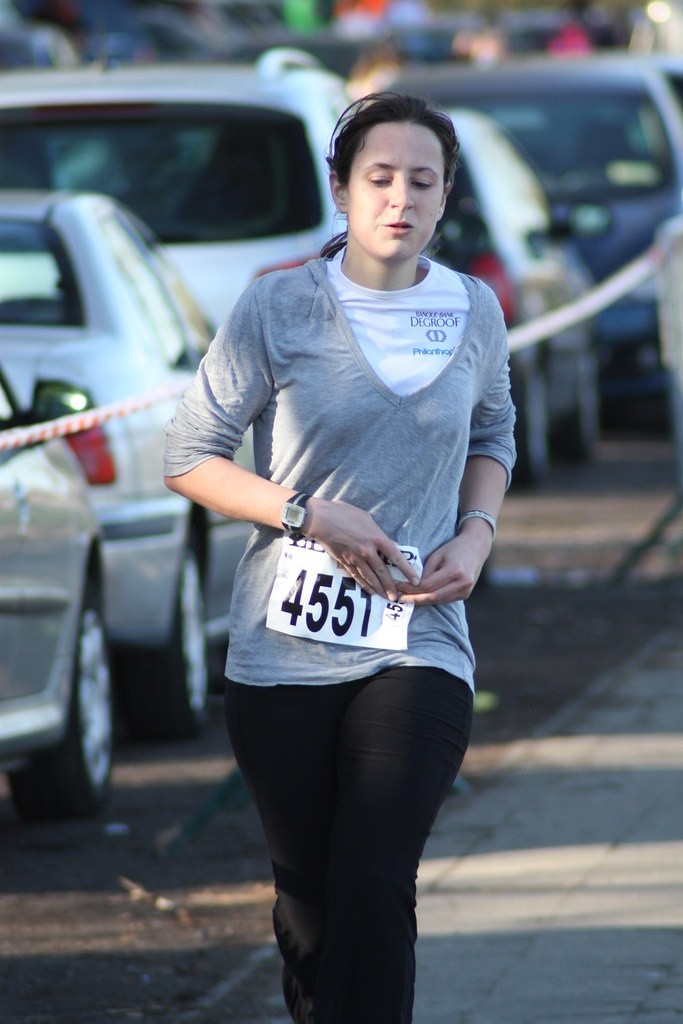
[0,47,348,334]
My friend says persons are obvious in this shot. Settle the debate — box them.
[163,91,517,1023]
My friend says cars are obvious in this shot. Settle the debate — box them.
[360,42,683,500]
[1,356,118,823]
[1,184,251,744]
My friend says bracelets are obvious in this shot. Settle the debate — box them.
[457,510,496,540]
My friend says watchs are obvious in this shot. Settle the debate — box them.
[281,492,312,539]
